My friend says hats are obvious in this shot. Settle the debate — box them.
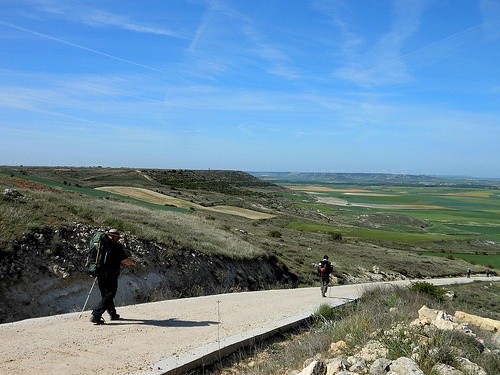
[108,229,121,238]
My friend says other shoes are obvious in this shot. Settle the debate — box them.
[322,291,326,297]
[90,316,104,324]
[110,314,119,320]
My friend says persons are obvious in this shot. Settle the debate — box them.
[317,255,333,296]
[83,229,127,320]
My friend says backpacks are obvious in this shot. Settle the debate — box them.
[86,231,111,277]
[318,260,328,277]
